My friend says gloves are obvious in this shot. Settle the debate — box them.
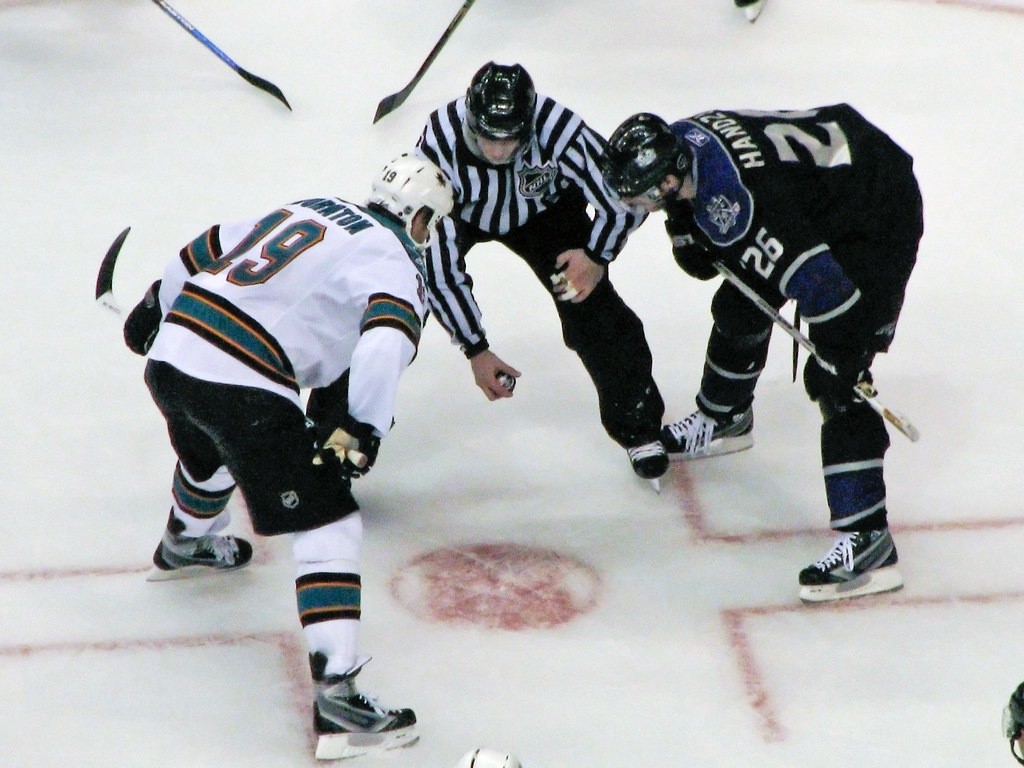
[834,354,879,403]
[665,217,722,280]
[123,279,162,357]
[311,412,381,482]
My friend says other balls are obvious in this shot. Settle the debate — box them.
[495,371,517,393]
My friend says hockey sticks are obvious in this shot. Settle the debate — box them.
[94,222,369,473]
[150,0,294,115]
[710,256,921,444]
[371,0,478,125]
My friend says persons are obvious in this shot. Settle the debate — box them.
[306,60,669,495]
[600,102,925,605]
[1001,681,1024,767]
[122,152,454,759]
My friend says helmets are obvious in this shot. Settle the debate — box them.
[1002,682,1024,766]
[455,749,522,768]
[365,152,454,252]
[601,112,690,217]
[462,61,535,165]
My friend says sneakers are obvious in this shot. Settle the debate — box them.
[659,404,755,461]
[145,506,253,580]
[621,417,669,495]
[308,650,420,759]
[798,526,904,602]
